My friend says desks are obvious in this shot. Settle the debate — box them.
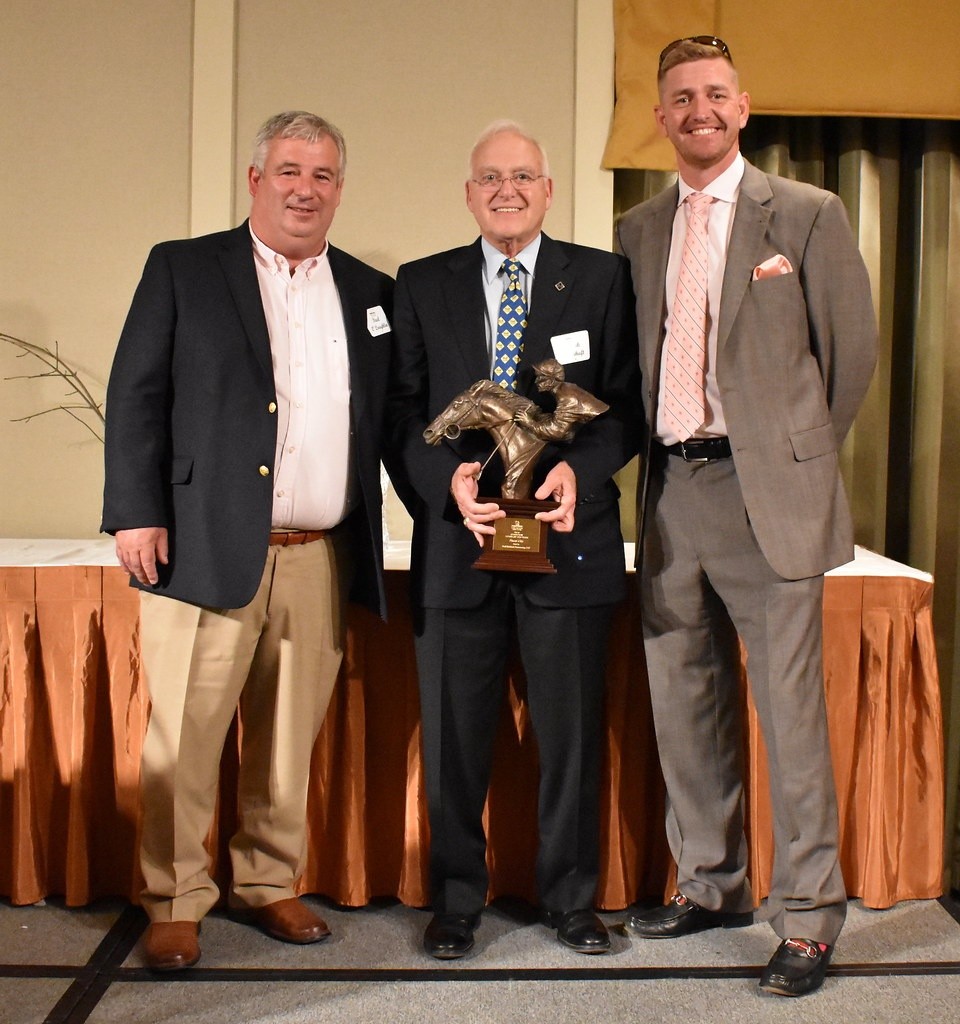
[0,539,947,912]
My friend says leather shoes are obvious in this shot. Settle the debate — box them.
[758,937,832,997]
[144,916,202,976]
[624,892,754,939]
[424,909,483,958]
[227,898,329,944]
[542,907,609,952]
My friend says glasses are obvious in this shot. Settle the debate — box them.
[472,174,542,190]
[658,35,732,74]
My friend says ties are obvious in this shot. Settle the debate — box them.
[663,193,714,443]
[494,259,527,394]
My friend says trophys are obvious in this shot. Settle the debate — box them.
[424,359,611,572]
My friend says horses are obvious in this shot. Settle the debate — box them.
[423,380,546,499]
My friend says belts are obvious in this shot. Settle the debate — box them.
[656,437,734,463]
[270,529,326,547]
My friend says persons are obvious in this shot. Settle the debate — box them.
[101,111,396,972]
[612,44,878,994]
[372,121,646,960]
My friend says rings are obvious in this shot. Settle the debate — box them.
[463,518,470,526]
